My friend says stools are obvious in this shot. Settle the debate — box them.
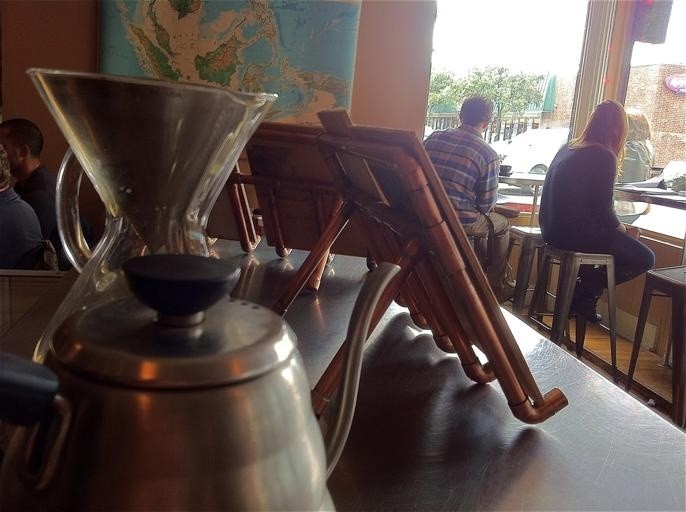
[504,225,554,323]
[624,264,686,430]
[527,246,616,367]
[467,234,517,304]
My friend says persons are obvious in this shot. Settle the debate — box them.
[536,98,656,325]
[0,140,45,268]
[420,94,519,306]
[610,109,656,228]
[0,115,77,273]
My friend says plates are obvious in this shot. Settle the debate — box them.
[633,182,660,187]
[499,173,513,176]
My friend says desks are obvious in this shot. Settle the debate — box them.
[0,238,686,512]
[498,173,686,211]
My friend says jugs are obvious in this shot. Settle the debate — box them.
[24,66,278,364]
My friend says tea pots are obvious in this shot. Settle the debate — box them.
[1,253,402,512]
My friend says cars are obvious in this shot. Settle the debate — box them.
[489,128,569,196]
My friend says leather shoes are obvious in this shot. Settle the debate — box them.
[491,280,530,304]
[568,276,604,324]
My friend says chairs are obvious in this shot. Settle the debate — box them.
[15,210,92,272]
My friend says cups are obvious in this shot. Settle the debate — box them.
[500,164,512,173]
